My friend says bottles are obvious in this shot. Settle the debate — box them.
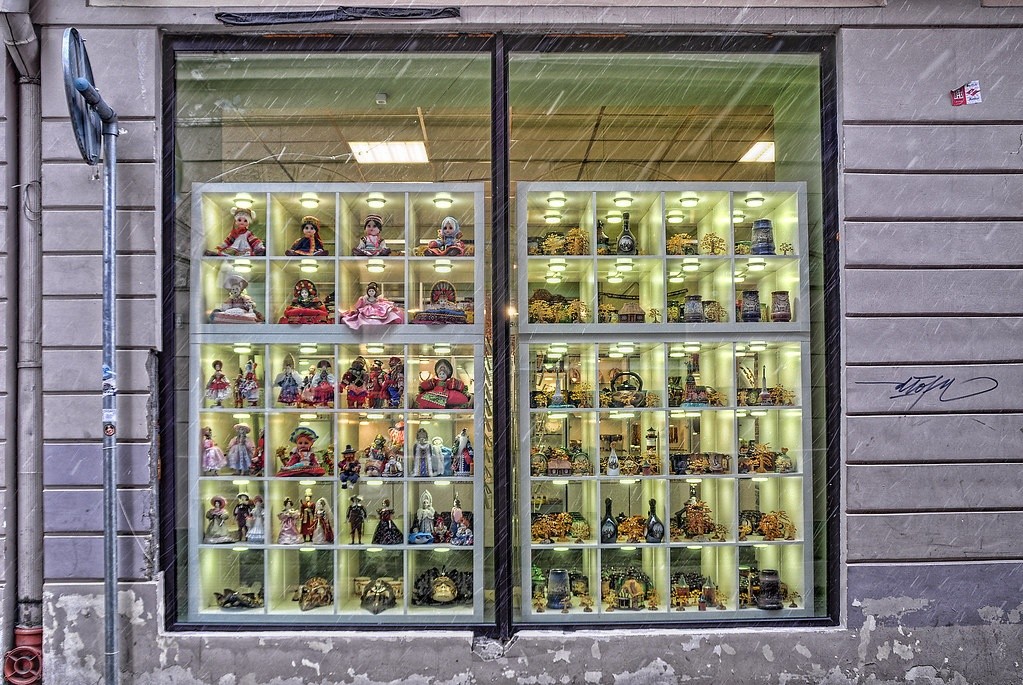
[667,295,719,323]
[546,568,572,609]
[553,379,564,406]
[600,499,619,543]
[667,376,684,407]
[759,365,770,401]
[641,499,666,543]
[702,575,715,606]
[751,219,776,255]
[606,448,620,476]
[616,213,636,255]
[735,291,791,321]
[758,569,784,610]
[596,220,610,255]
[676,575,689,599]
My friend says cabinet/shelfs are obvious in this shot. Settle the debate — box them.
[188,182,485,624]
[516,181,814,622]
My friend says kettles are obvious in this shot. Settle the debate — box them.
[603,372,645,407]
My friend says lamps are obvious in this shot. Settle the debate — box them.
[233,192,455,352]
[545,191,767,359]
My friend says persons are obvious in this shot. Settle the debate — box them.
[284,216,329,256]
[339,282,404,328]
[276,487,335,543]
[371,498,404,545]
[208,276,264,324]
[423,216,464,255]
[412,360,470,409]
[199,422,266,479]
[203,492,265,545]
[214,206,266,256]
[275,427,327,477]
[337,419,406,489]
[205,358,260,408]
[410,489,475,546]
[277,280,329,323]
[351,214,391,256]
[345,494,367,544]
[412,427,475,477]
[272,353,337,409]
[340,356,405,408]
